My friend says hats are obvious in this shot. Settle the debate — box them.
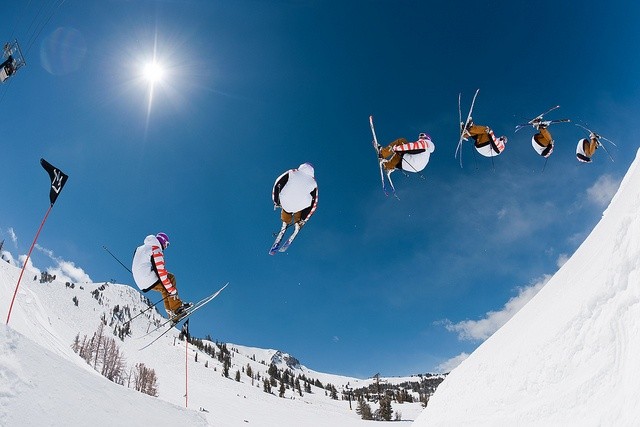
[425,133,431,139]
[156,232,169,245]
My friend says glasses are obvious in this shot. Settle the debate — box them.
[418,132,427,138]
[156,234,170,248]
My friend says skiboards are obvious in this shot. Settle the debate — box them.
[369,115,401,202]
[268,221,300,255]
[575,122,617,166]
[454,89,480,169]
[513,105,571,132]
[137,281,230,350]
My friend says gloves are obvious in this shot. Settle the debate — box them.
[298,219,306,228]
[168,288,179,300]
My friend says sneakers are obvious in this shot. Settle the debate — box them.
[460,121,465,130]
[172,309,191,322]
[539,123,550,128]
[594,133,601,139]
[378,143,383,159]
[535,117,541,122]
[592,138,600,145]
[170,301,194,317]
[466,116,474,131]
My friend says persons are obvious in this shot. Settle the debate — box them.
[271,162,319,227]
[377,131,436,177]
[460,116,507,157]
[575,132,601,162]
[131,232,194,325]
[531,117,555,157]
